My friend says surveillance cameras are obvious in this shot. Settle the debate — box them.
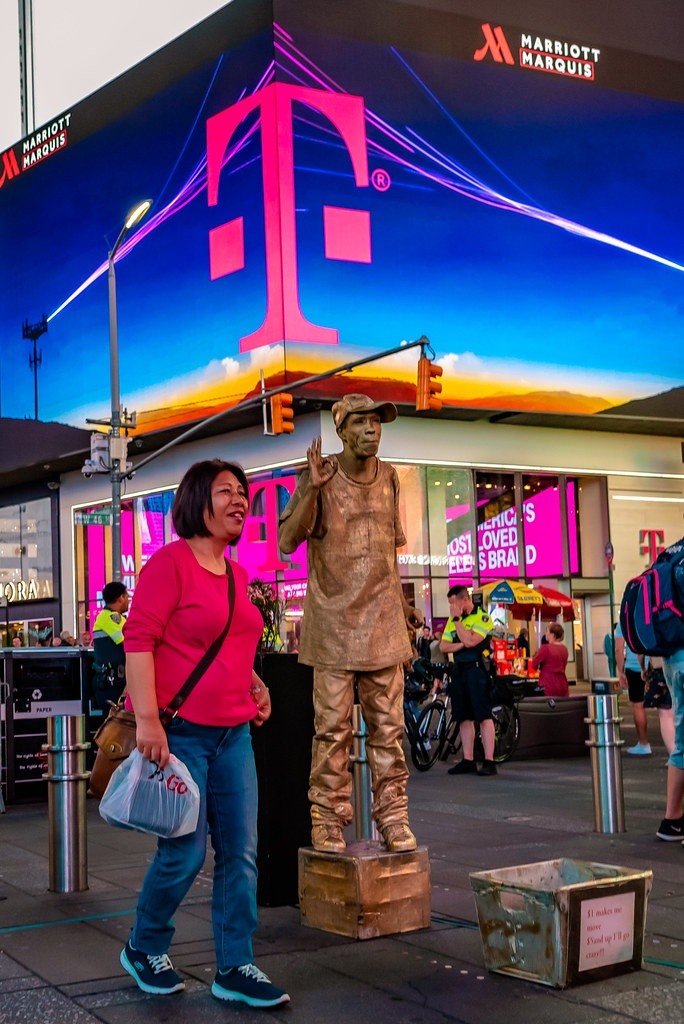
[127,471,136,480]
[81,465,95,478]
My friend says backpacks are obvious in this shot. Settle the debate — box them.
[620,537,684,657]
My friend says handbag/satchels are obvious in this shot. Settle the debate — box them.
[88,708,171,798]
[97,745,199,838]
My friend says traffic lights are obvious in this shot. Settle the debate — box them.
[270,392,295,435]
[416,357,443,412]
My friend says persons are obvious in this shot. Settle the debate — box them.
[119,459,290,1008]
[93,581,129,717]
[417,626,445,696]
[439,585,498,775]
[532,623,569,697]
[279,393,424,852]
[615,621,676,766]
[12,630,91,648]
[510,628,531,670]
[650,536,684,841]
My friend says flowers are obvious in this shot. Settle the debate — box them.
[247,578,288,653]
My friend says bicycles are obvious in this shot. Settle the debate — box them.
[403,657,528,772]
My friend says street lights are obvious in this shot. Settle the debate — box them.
[108,197,154,582]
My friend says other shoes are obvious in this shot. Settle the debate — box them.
[626,742,652,754]
[448,759,477,774]
[476,759,497,777]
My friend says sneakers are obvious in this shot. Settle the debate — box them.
[119,938,185,994]
[211,963,290,1007]
[656,816,684,841]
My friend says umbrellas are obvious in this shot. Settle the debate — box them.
[474,578,579,649]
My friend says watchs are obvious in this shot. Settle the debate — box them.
[451,616,460,622]
